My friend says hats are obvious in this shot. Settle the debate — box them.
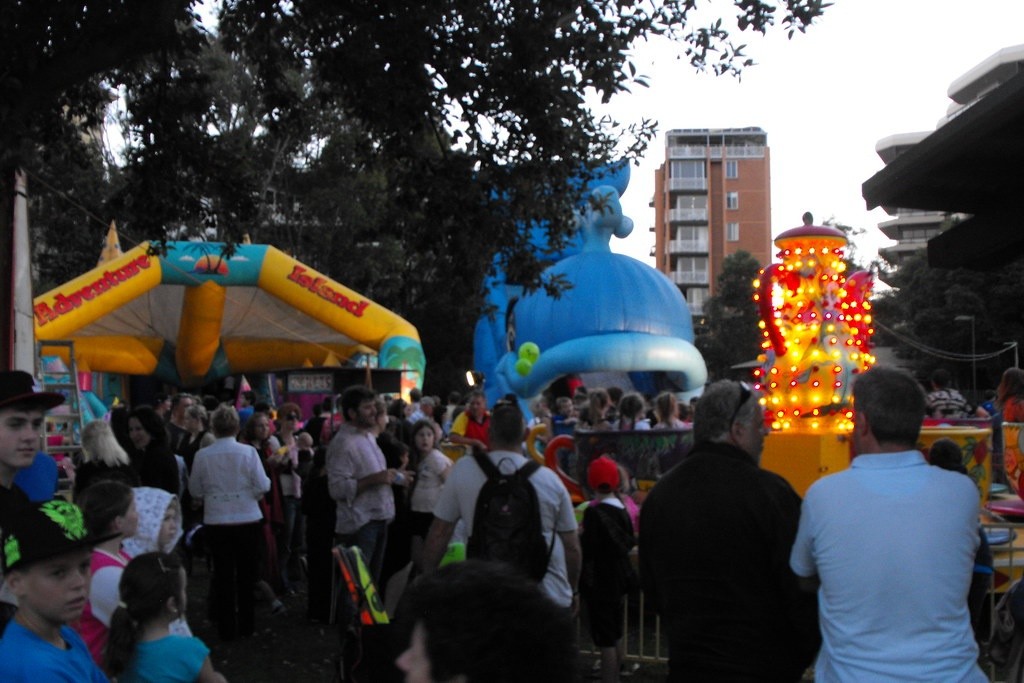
[0,370,66,411]
[588,456,620,494]
[0,495,122,574]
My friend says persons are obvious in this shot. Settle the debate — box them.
[927,436,991,643]
[787,366,991,683]
[190,386,417,642]
[994,368,1024,423]
[923,369,974,418]
[418,388,701,683]
[394,557,584,683]
[0,370,226,683]
[638,379,824,683]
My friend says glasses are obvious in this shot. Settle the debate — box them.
[727,381,751,435]
[282,414,300,421]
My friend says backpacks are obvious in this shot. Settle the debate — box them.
[466,448,556,584]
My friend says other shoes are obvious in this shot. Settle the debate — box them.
[266,599,288,620]
[587,659,634,677]
[275,585,296,597]
[306,610,340,626]
